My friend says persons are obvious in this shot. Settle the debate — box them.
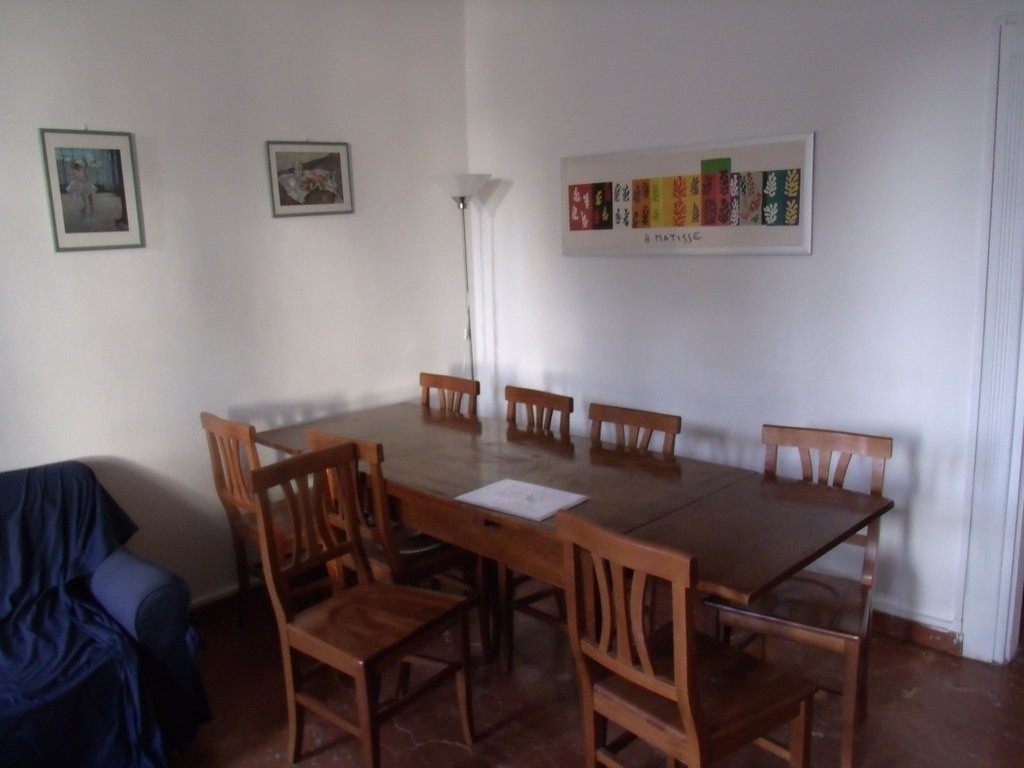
[66,158,97,215]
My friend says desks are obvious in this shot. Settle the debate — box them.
[254,401,894,646]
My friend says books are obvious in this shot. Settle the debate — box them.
[454,478,589,522]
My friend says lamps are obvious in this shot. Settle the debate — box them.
[433,173,492,382]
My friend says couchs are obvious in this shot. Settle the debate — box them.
[0,460,216,768]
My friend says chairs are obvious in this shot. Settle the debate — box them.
[497,402,682,670]
[700,425,894,768]
[200,412,494,768]
[499,385,574,438]
[555,510,820,768]
[418,371,479,422]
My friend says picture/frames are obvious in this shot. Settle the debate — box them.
[265,140,354,219]
[37,128,146,253]
[560,130,816,259]
